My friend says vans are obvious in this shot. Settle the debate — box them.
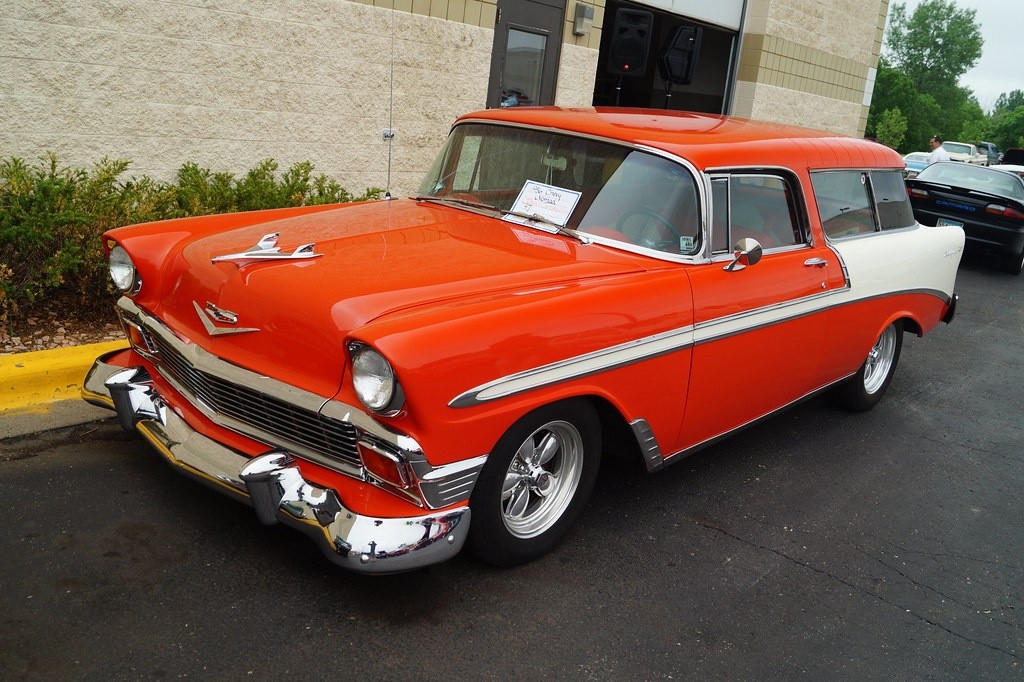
[977,142,1000,166]
[1002,148,1024,165]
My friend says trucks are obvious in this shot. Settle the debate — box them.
[941,141,987,173]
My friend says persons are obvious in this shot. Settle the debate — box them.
[929,135,951,164]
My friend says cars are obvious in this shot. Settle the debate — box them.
[904,150,931,177]
[905,161,1024,277]
[80,105,969,583]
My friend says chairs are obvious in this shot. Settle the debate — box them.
[536,157,872,259]
